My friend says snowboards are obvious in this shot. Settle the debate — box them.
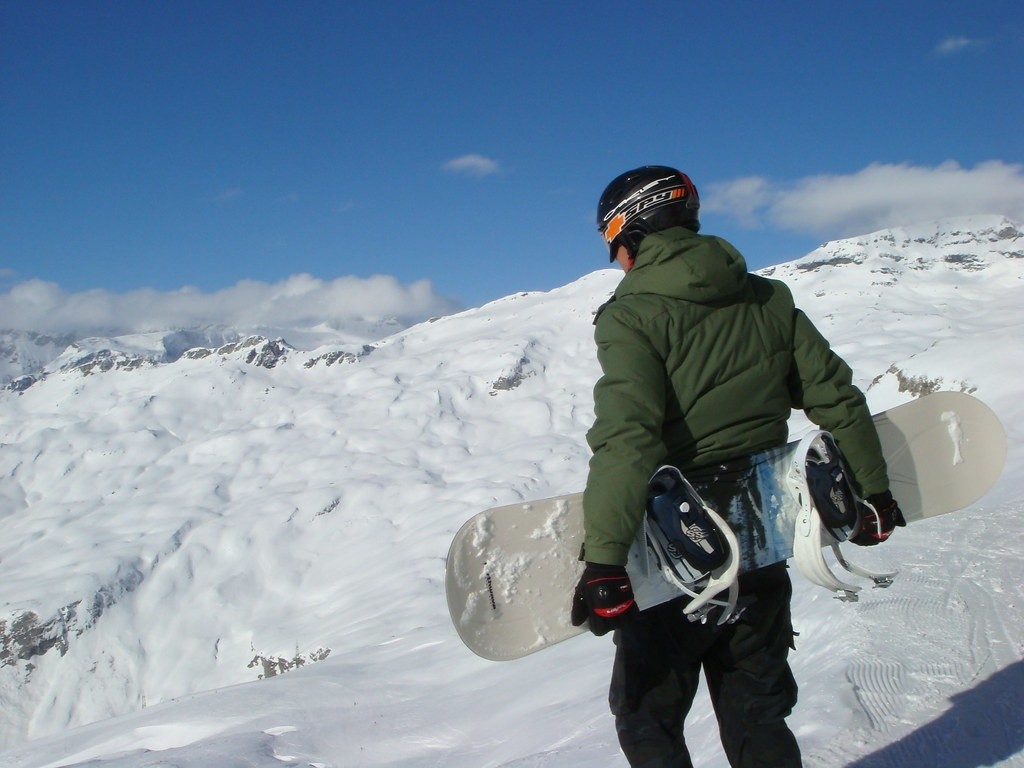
[443,386,1009,668]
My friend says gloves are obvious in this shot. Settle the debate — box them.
[570,563,640,636]
[848,493,906,547]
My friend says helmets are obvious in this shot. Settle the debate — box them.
[596,165,700,263]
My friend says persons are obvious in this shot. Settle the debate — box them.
[569,165,905,768]
